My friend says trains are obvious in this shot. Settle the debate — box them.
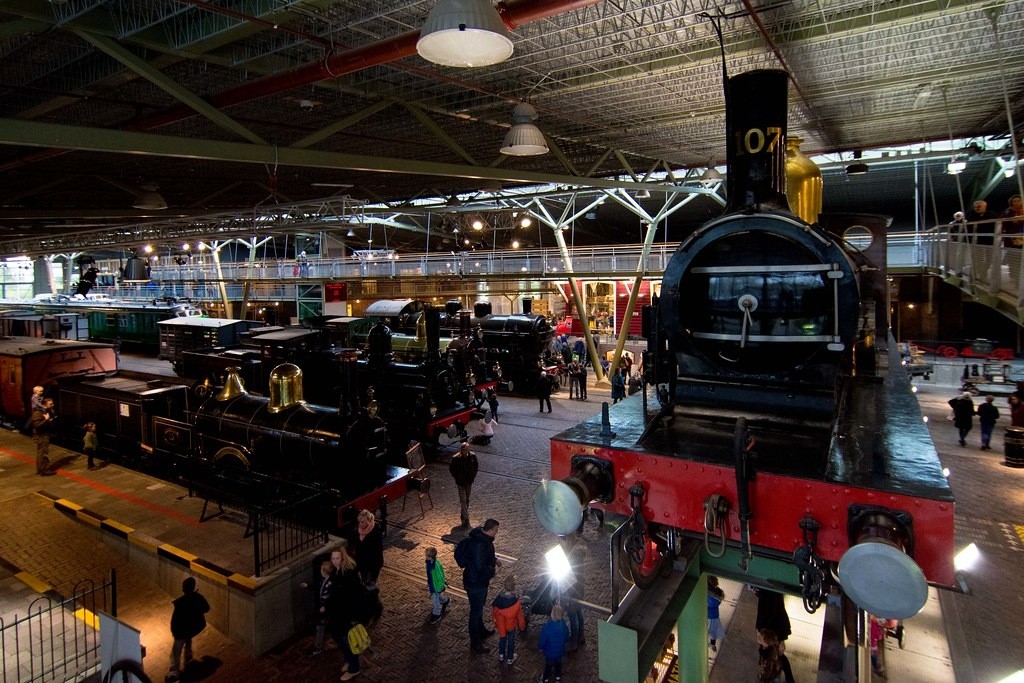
[0,335,431,531]
[1,293,210,347]
[528,67,953,618]
[172,309,492,451]
[301,298,562,399]
[0,309,89,341]
[320,308,514,402]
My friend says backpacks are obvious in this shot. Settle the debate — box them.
[347,624,371,655]
[454,536,491,568]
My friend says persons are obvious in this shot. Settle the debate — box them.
[462,518,497,655]
[32,386,58,421]
[492,575,527,665]
[755,625,795,683]
[170,577,211,671]
[489,394,499,422]
[536,371,553,413]
[611,350,645,404]
[45,329,53,339]
[752,586,792,655]
[948,394,1024,450]
[81,422,100,470]
[536,605,569,683]
[425,547,451,624]
[298,509,386,682]
[449,442,478,527]
[706,575,725,652]
[561,342,588,402]
[871,615,890,677]
[31,398,57,477]
[297,251,308,277]
